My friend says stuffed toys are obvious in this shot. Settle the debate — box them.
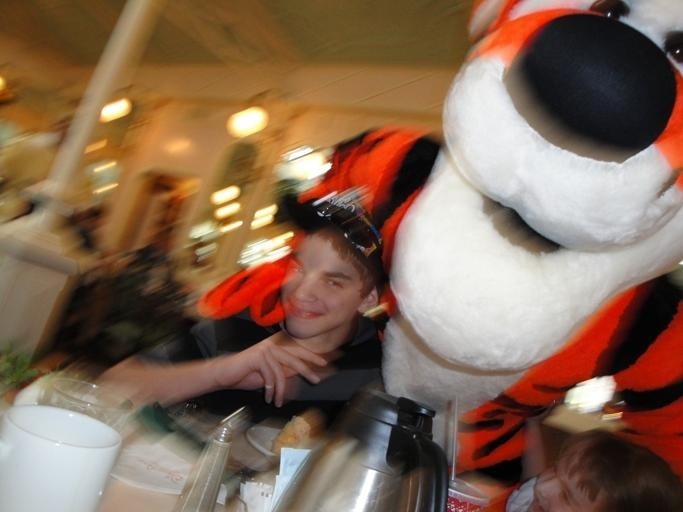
[198,0,683,484]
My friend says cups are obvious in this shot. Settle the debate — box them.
[42,376,136,425]
[448,476,489,512]
[1,404,124,512]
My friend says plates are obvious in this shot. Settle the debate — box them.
[245,421,322,458]
[108,443,192,496]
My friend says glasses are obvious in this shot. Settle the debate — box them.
[308,198,383,259]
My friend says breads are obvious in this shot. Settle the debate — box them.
[271,406,325,456]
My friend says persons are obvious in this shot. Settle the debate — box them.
[506,403,683,512]
[93,189,383,441]
